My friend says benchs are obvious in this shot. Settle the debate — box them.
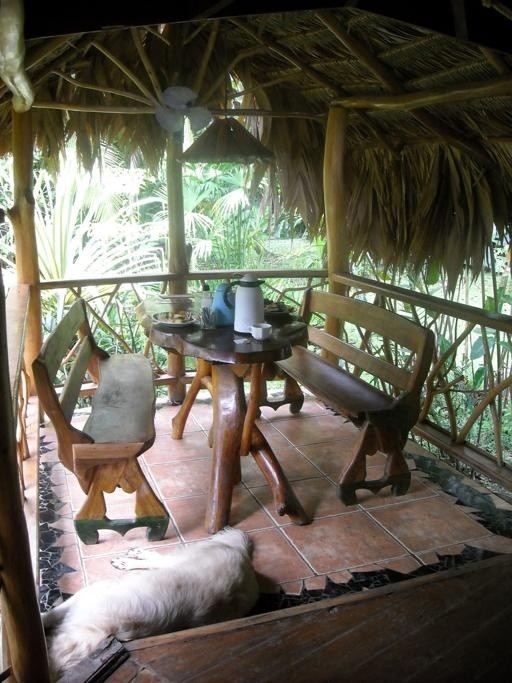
[256,288,434,506]
[32,299,169,545]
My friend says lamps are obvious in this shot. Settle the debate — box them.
[180,34,275,163]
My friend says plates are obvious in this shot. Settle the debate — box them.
[153,311,197,328]
[263,303,297,316]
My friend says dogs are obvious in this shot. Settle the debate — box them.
[41,525,255,682]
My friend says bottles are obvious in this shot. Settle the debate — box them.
[212,283,237,326]
[202,284,214,308]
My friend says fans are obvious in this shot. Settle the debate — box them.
[155,85,212,132]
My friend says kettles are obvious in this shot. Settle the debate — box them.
[224,273,266,336]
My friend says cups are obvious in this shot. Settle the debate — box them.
[199,310,216,328]
[247,323,274,340]
